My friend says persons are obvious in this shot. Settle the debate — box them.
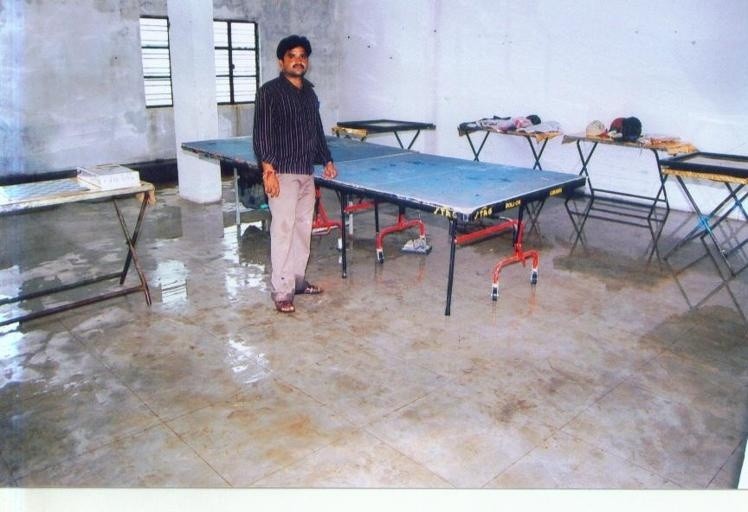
[252,34,337,312]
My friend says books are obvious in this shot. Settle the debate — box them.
[76,160,142,190]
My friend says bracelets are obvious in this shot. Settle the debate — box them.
[262,170,276,177]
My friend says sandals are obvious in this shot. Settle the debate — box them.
[275,300,295,312]
[295,283,323,294]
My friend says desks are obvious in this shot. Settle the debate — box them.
[180,134,588,317]
[561,131,677,219]
[657,149,748,276]
[1,177,157,327]
[336,117,437,232]
[457,120,565,240]
